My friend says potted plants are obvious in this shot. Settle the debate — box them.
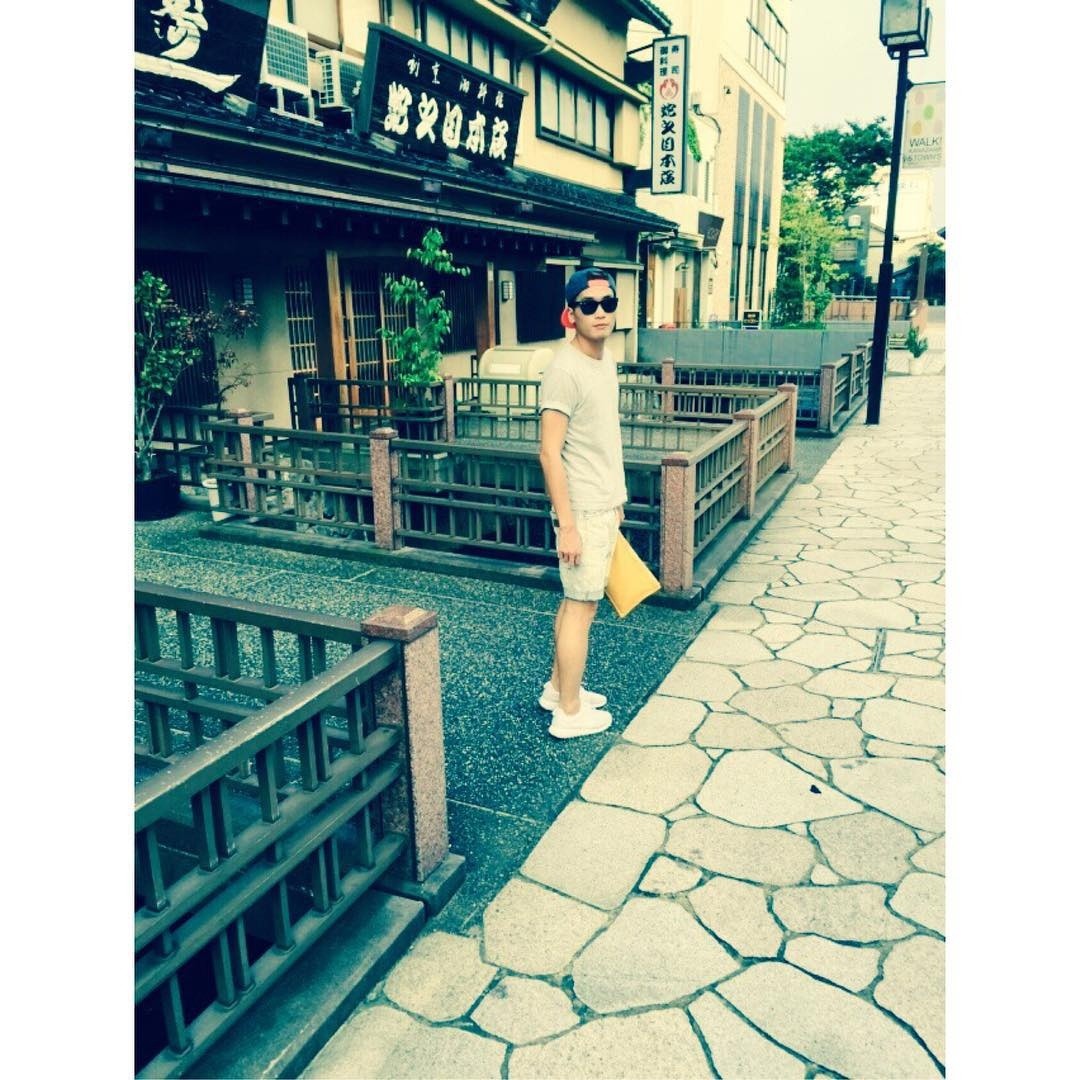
[374,228,472,455]
[135,270,202,521]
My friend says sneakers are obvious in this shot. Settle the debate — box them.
[538,681,607,711]
[548,702,612,738]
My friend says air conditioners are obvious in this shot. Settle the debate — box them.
[259,17,310,98]
[315,50,365,108]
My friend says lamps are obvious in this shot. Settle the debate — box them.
[420,177,443,196]
[502,281,513,300]
[513,202,533,215]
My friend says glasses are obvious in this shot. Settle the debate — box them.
[570,297,618,315]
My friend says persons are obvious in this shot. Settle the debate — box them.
[535,267,630,742]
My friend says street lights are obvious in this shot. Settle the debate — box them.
[864,2,935,427]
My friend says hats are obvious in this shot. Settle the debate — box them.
[561,268,616,328]
